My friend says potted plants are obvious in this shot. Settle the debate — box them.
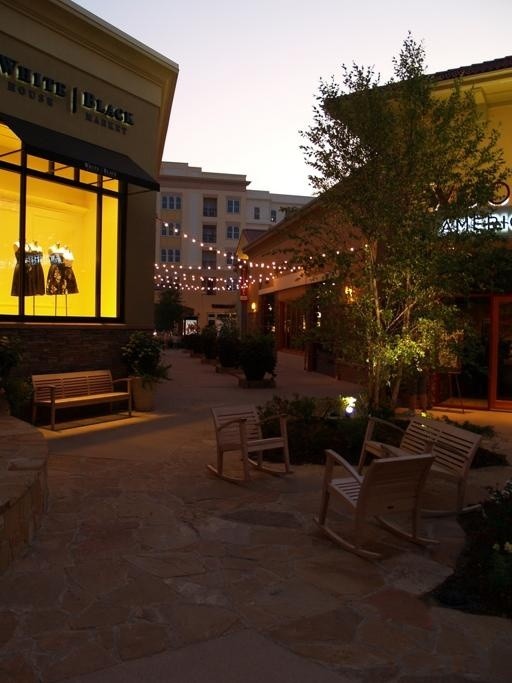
[120,329,173,412]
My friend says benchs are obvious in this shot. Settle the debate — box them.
[31,368,132,429]
[357,416,483,518]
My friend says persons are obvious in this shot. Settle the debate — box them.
[29,241,45,295]
[64,247,79,293]
[11,243,36,296]
[46,243,68,295]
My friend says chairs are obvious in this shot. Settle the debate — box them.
[206,402,296,483]
[311,441,440,561]
